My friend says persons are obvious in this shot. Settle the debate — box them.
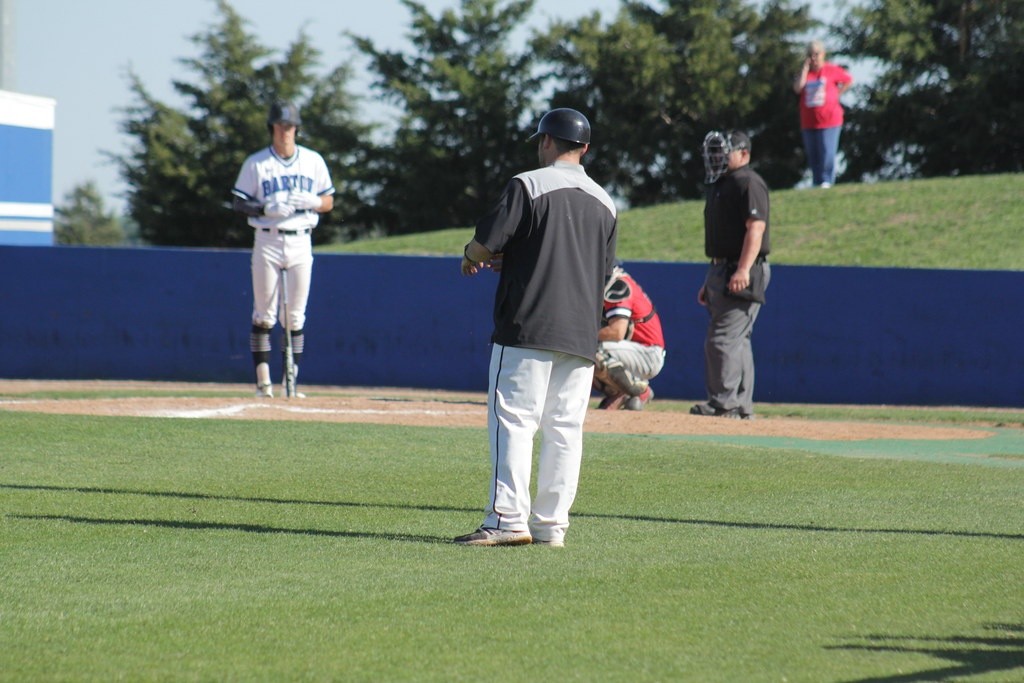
[591,264,666,410]
[689,127,772,419]
[231,100,336,399]
[793,39,855,192]
[455,109,617,547]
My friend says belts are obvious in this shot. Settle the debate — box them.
[711,256,765,266]
[262,228,310,234]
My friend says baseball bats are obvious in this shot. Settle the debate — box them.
[281,268,296,398]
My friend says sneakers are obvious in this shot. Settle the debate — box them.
[600,392,629,410]
[453,527,532,546]
[532,537,564,547]
[627,387,654,410]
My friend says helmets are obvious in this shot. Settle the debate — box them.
[266,101,301,135]
[525,109,589,145]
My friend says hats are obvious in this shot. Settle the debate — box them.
[722,130,752,152]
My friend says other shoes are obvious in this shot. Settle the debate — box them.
[690,404,720,415]
[719,408,748,420]
[281,392,304,398]
[256,391,273,399]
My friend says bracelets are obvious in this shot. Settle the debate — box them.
[463,243,479,266]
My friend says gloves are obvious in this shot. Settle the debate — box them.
[263,201,294,217]
[288,189,322,210]
